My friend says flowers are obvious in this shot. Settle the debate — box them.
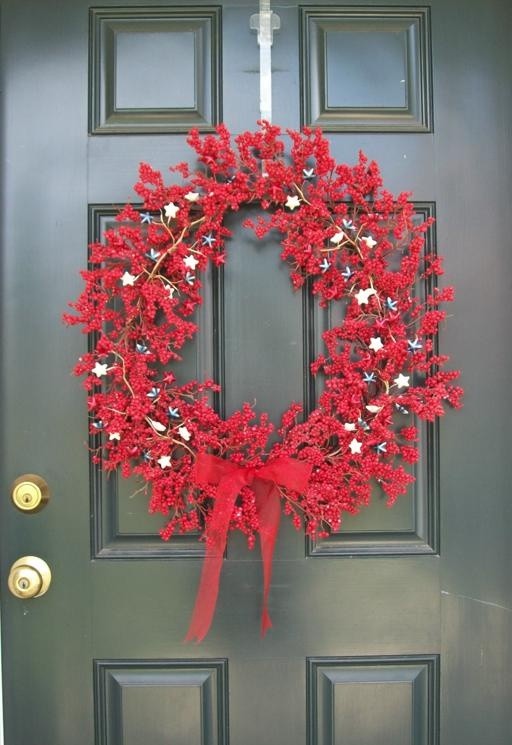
[57,117,469,559]
